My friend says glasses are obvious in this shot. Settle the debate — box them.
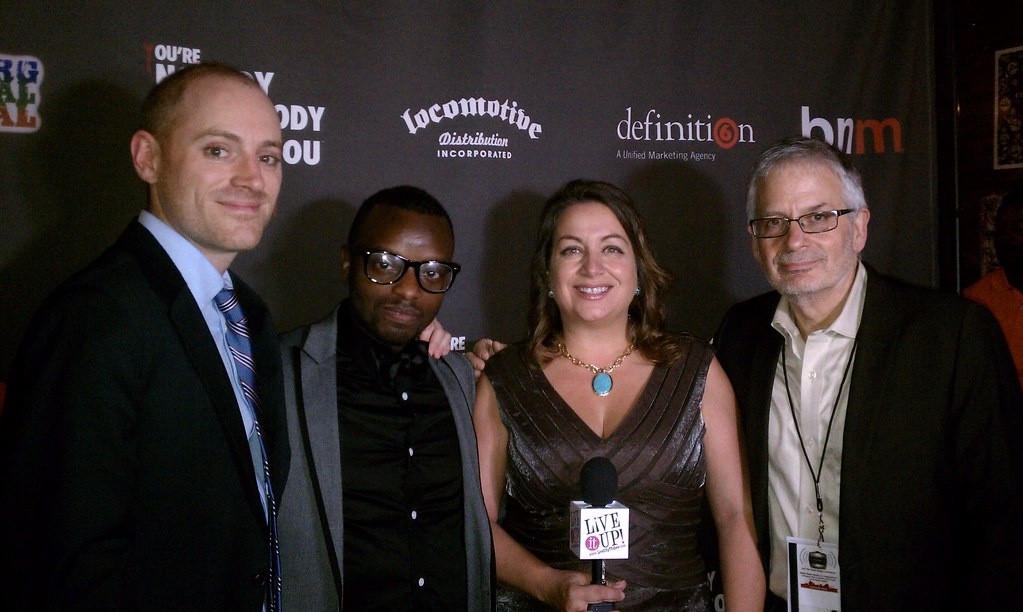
[749,208,857,238]
[353,249,460,294]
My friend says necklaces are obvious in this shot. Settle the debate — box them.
[558,343,633,397]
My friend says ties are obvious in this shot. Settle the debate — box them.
[215,289,282,612]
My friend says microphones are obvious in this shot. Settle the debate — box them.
[570,456,629,612]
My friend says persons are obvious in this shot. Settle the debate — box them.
[0,63,451,612]
[274,185,497,612]
[962,192,1023,384]
[467,136,1023,612]
[472,179,767,612]
